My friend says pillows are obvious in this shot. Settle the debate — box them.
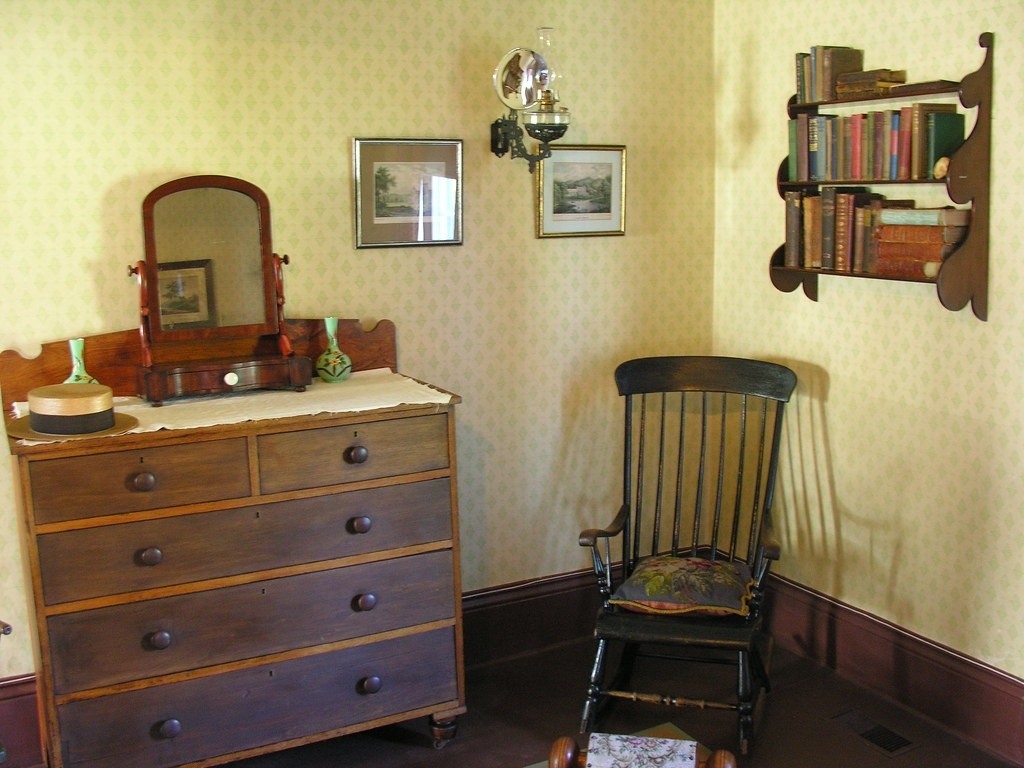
[608,555,754,617]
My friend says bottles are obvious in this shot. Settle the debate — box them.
[316,315,352,385]
[62,338,99,384]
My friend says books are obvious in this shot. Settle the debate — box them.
[788,45,969,283]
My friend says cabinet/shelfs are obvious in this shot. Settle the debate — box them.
[768,31,995,322]
[2,396,467,768]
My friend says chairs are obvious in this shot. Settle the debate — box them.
[578,355,798,765]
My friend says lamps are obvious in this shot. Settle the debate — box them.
[490,28,571,174]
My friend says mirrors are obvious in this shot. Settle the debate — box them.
[142,174,280,343]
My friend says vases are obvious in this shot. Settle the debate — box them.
[62,337,101,384]
[316,317,352,383]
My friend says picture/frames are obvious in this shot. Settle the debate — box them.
[350,136,465,249]
[157,258,218,330]
[531,144,629,240]
